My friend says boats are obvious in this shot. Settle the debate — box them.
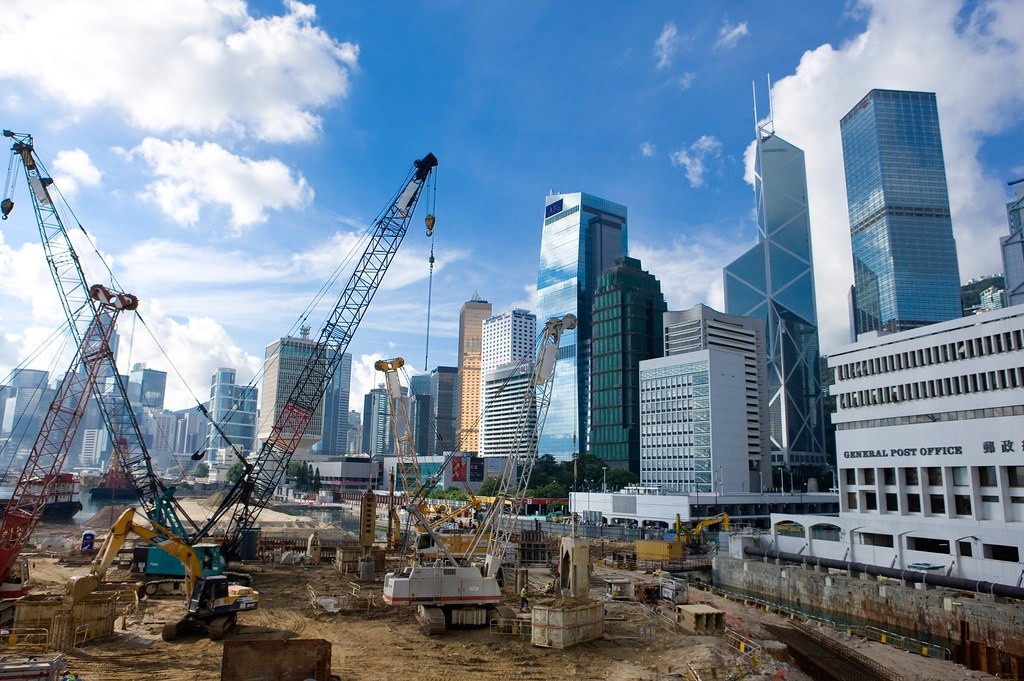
[86,436,144,501]
[1,473,83,520]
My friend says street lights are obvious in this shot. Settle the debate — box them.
[718,466,724,497]
[790,473,793,495]
[759,472,762,494]
[779,468,783,496]
[602,466,607,493]
[831,470,835,488]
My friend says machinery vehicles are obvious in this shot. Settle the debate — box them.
[64,505,260,641]
[1,284,140,624]
[384,312,578,639]
[372,355,495,564]
[675,512,731,557]
[1,130,254,599]
